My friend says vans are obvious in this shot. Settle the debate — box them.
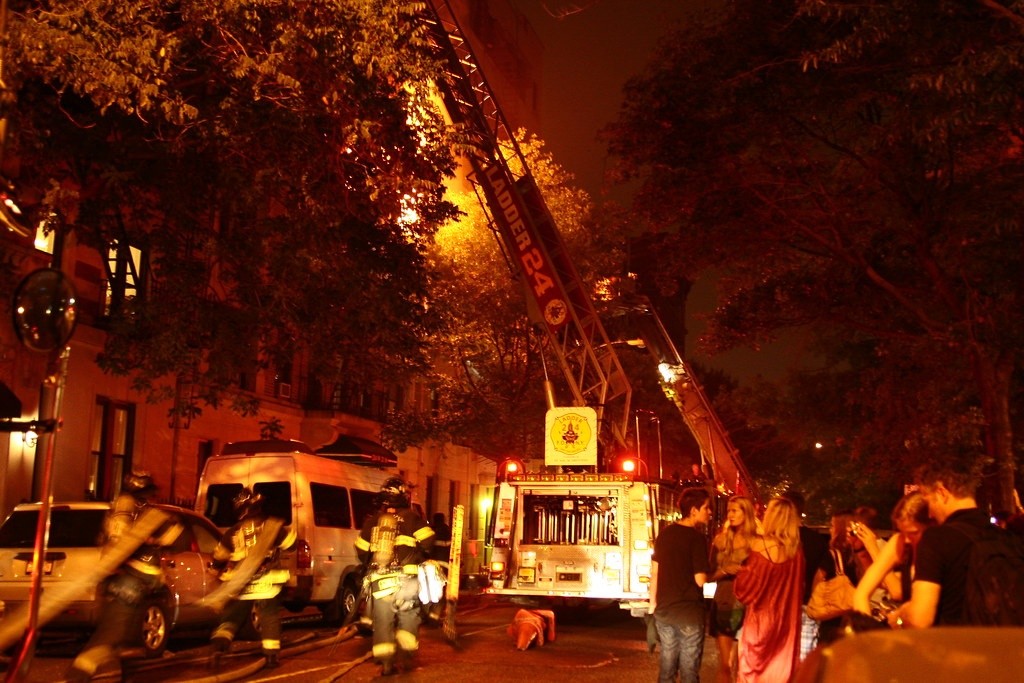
[194,434,429,627]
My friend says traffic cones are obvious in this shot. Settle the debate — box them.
[506,608,556,651]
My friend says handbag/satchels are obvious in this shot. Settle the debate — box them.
[807,548,857,620]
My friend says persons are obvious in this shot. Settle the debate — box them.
[203,489,299,673]
[353,478,451,675]
[65,459,193,683]
[647,489,805,683]
[802,452,1024,648]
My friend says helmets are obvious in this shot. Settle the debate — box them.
[123,470,160,498]
[382,478,408,503]
[233,491,261,519]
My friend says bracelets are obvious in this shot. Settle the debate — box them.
[896,607,908,630]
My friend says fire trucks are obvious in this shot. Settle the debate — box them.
[396,0,895,617]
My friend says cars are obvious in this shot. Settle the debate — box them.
[0,501,262,659]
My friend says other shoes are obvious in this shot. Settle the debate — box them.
[382,661,398,675]
[208,646,222,673]
[403,657,418,670]
[265,655,279,668]
